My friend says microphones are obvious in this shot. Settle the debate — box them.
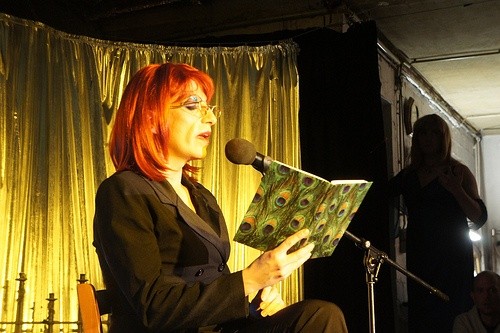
[225,139,273,173]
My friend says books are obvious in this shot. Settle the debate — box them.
[233,160,374,260]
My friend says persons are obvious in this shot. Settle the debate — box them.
[93,62,348,333]
[452,271,500,333]
[387,115,487,333]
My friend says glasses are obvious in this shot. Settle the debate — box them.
[169,95,222,123]
